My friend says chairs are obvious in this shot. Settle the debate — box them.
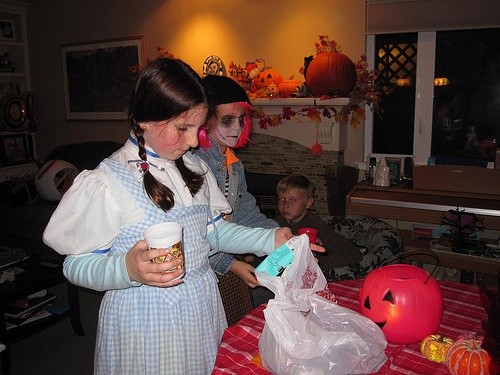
[325,214,401,284]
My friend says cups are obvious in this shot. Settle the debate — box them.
[297,227,318,245]
[143,222,186,283]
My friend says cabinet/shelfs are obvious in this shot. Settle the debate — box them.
[0,0,39,181]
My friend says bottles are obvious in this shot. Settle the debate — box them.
[369,157,377,186]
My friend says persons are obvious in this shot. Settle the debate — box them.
[42,57,325,375]
[188,76,363,310]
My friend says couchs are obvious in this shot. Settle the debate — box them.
[0,139,123,295]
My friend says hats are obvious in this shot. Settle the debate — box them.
[202,75,250,109]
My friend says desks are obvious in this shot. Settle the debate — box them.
[211,278,500,375]
[398,227,500,284]
[0,245,85,375]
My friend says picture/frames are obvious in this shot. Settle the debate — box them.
[59,36,145,120]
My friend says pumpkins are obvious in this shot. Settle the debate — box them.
[445,339,500,375]
[279,75,303,97]
[420,335,454,363]
[306,46,357,96]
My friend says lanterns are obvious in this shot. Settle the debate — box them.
[355,264,442,344]
[251,52,359,98]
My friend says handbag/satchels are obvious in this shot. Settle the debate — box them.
[253,237,337,304]
[257,288,387,375]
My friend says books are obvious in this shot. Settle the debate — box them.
[4,293,58,330]
